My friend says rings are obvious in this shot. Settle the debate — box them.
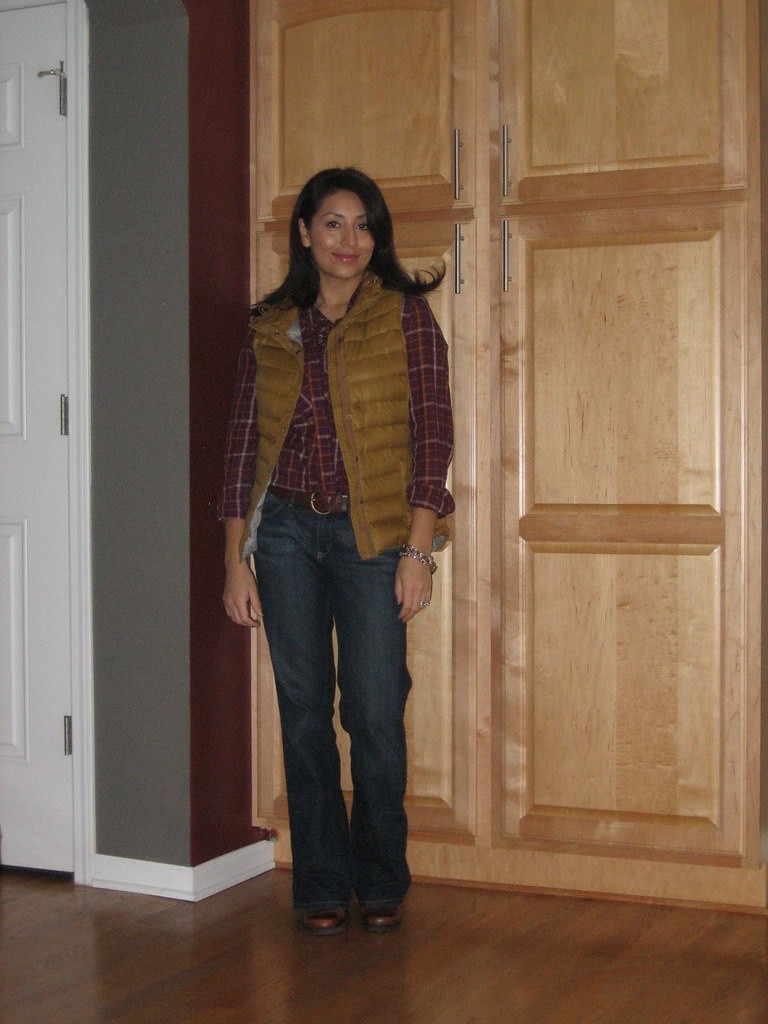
[420,600,430,607]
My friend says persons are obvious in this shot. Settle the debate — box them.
[217,167,455,933]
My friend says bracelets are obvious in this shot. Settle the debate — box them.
[398,543,437,574]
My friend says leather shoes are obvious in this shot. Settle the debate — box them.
[363,897,405,932]
[300,906,349,933]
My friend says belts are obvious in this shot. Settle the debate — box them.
[267,483,351,515]
